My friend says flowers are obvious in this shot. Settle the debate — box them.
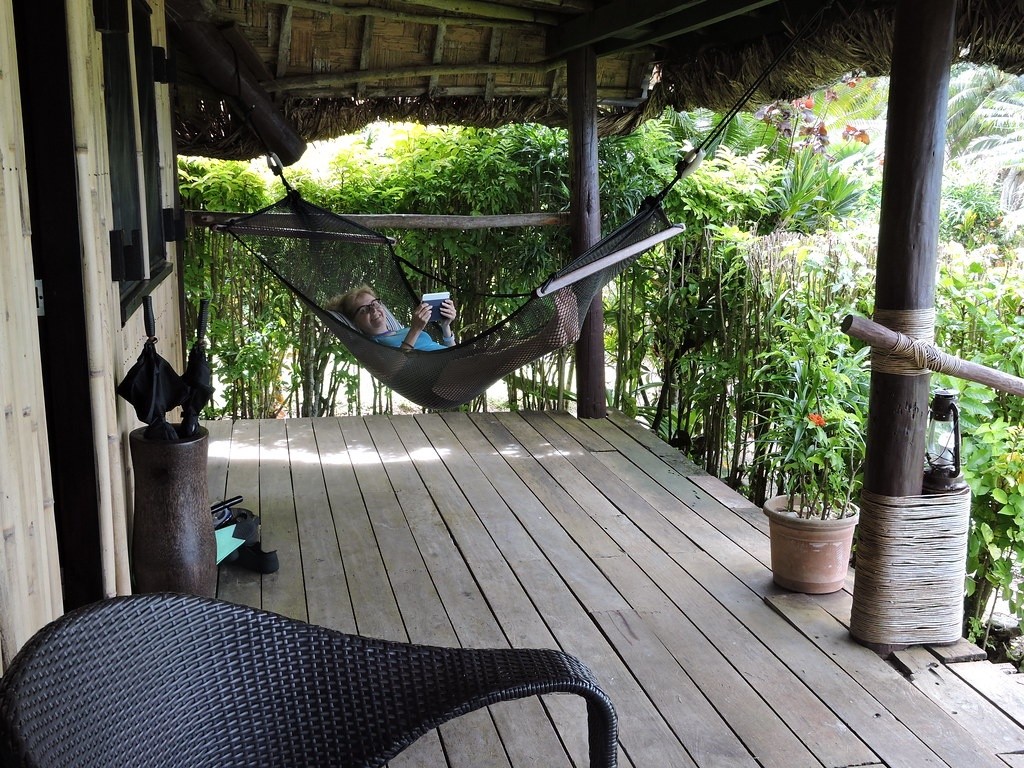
[750,308,870,518]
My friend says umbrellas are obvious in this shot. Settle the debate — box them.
[180,300,217,441]
[116,296,190,441]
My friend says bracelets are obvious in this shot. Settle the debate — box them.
[401,341,414,349]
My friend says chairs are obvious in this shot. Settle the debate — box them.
[0,592,619,768]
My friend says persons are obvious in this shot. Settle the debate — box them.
[322,285,581,410]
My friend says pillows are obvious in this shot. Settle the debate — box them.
[326,304,403,344]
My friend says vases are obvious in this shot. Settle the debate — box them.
[127,422,218,599]
[760,493,860,594]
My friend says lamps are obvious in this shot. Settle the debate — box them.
[923,388,971,496]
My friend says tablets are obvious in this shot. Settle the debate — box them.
[422,292,451,321]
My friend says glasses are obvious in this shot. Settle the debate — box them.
[354,298,381,316]
